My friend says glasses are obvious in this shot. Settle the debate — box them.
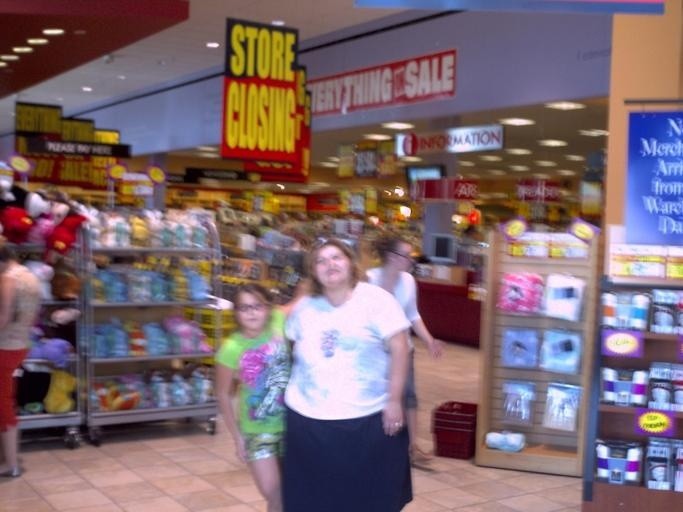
[388,248,414,263]
[230,303,266,311]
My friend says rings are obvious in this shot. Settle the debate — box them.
[394,422,399,426]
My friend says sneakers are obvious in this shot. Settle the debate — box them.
[408,447,434,465]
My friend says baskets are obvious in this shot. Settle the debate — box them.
[429,399,477,461]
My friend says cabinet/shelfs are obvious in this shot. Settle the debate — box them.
[0,227,83,453]
[473,227,597,478]
[579,272,683,511]
[82,215,222,449]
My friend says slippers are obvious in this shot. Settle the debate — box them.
[0,465,20,478]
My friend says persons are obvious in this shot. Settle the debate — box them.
[360,235,446,465]
[278,237,413,511]
[0,246,42,478]
[212,281,314,512]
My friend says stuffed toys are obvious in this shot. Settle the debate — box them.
[0,178,217,420]
